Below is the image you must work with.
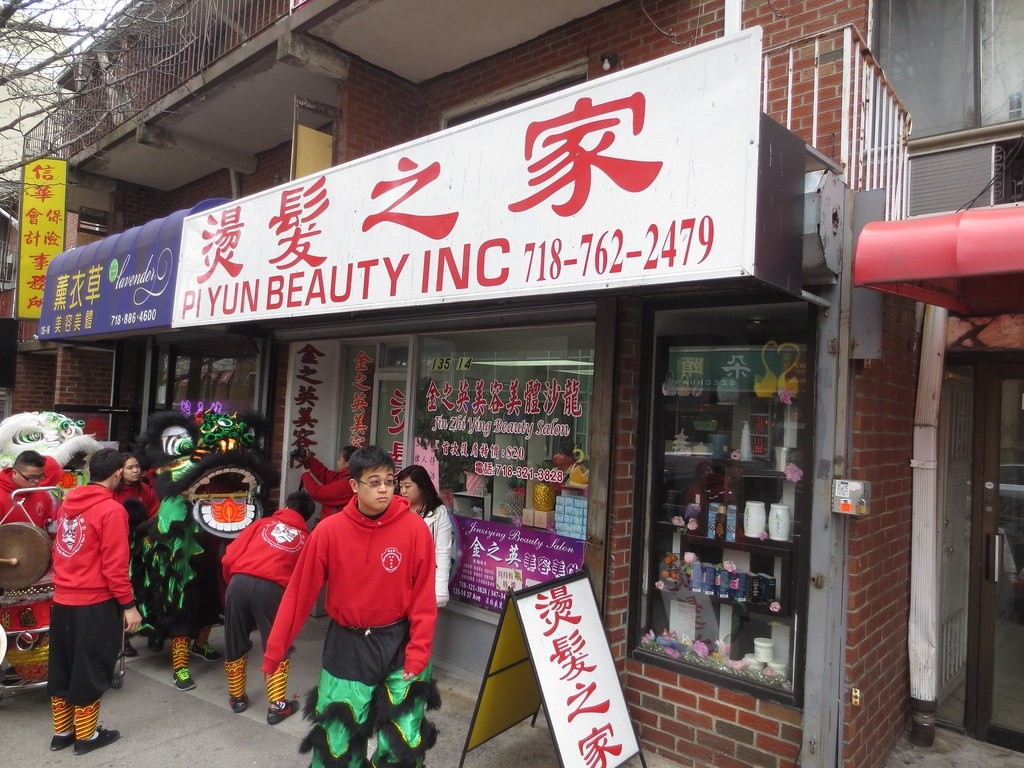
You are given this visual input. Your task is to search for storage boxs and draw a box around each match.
[523,496,588,540]
[690,560,775,606]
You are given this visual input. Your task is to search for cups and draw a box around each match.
[773,446,795,473]
[708,433,729,460]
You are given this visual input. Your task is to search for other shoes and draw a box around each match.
[122,642,138,657]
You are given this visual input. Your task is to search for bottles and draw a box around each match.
[743,501,766,538]
[740,420,752,462]
[744,638,787,678]
[768,503,791,540]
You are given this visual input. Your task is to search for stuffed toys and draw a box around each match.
[129,409,280,653]
[0,411,103,468]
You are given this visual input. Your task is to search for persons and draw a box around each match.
[0,450,53,528]
[50,448,143,755]
[221,491,316,723]
[260,445,443,768]
[169,625,223,690]
[301,446,358,521]
[113,452,151,505]
[686,447,746,563]
[397,465,452,607]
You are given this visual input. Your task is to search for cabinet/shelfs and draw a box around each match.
[453,430,590,525]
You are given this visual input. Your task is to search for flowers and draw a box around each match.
[671,513,697,534]
[759,532,768,541]
[764,667,775,677]
[731,449,740,460]
[770,601,781,612]
[654,388,801,685]
[785,462,803,482]
[654,552,736,592]
[731,659,749,671]
[639,627,708,659]
[778,388,793,405]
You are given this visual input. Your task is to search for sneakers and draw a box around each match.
[74,725,120,754]
[172,667,196,690]
[191,642,223,661]
[229,693,249,713]
[267,700,299,725]
[51,725,76,751]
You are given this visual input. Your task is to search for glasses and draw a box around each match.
[14,468,45,483]
[355,478,399,489]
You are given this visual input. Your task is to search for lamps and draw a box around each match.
[600,53,617,70]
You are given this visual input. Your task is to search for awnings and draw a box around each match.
[853,206,1024,317]
